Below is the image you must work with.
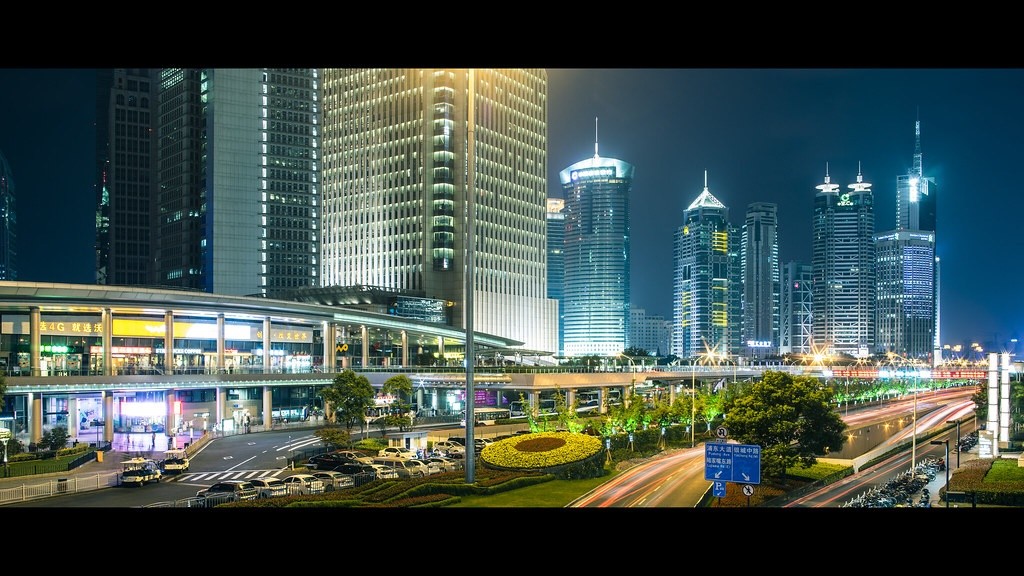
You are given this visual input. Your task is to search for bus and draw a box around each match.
[363,404,419,425]
[459,408,511,428]
[510,400,557,416]
[580,391,620,406]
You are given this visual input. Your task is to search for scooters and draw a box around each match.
[843,457,946,507]
[955,424,986,453]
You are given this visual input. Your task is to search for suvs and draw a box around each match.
[196,480,258,507]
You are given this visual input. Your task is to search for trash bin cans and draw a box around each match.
[94,451,104,463]
[57,478,68,493]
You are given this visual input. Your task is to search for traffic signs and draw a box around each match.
[705,443,761,483]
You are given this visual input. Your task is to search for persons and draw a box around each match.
[431,407,434,417]
[152,432,156,446]
[247,416,250,433]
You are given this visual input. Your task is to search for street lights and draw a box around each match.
[720,355,736,383]
[887,352,917,478]
[692,352,714,448]
[930,440,949,508]
[946,420,959,468]
[846,360,862,416]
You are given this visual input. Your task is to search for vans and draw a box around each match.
[372,457,421,479]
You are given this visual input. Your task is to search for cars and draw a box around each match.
[447,437,485,452]
[339,451,374,465]
[474,438,494,445]
[283,474,325,495]
[410,459,441,477]
[307,453,359,471]
[378,447,419,460]
[333,465,377,486]
[248,477,292,498]
[434,441,465,455]
[313,471,355,493]
[430,457,463,472]
[370,464,400,479]
